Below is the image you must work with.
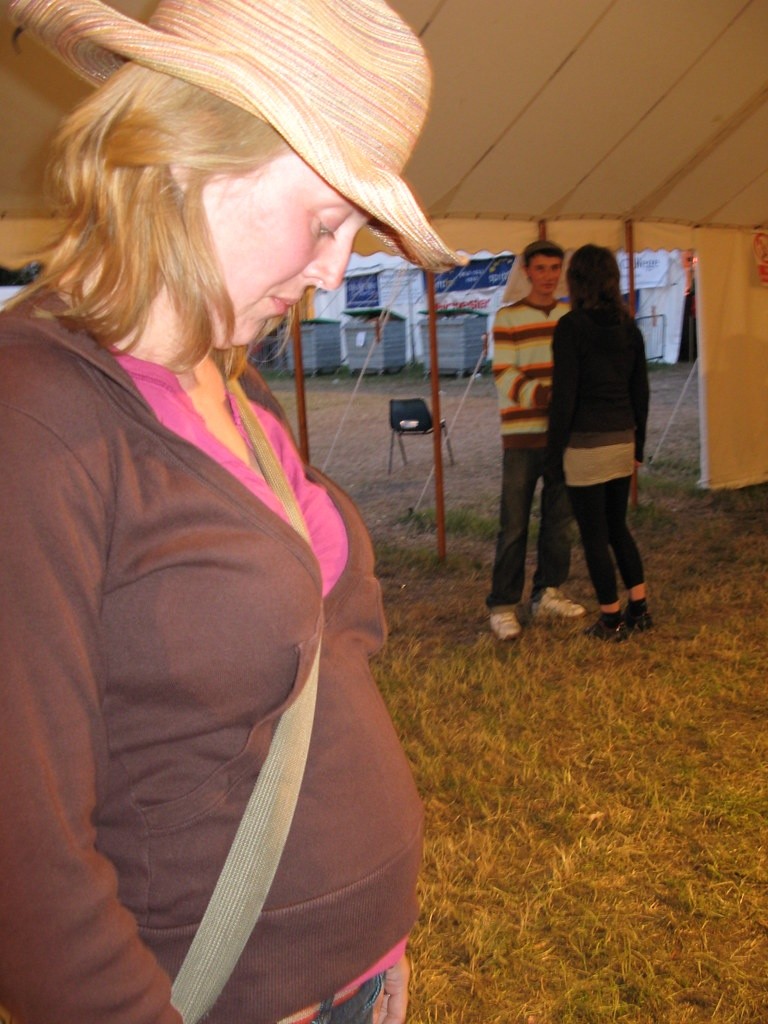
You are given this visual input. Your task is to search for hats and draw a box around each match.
[9,0,471,276]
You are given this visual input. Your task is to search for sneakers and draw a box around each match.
[531,588,585,620]
[491,613,521,639]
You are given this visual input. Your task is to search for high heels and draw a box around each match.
[587,619,628,644]
[622,610,652,630]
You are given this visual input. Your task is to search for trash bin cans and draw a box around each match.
[286,319,341,377]
[342,307,407,375]
[418,308,489,379]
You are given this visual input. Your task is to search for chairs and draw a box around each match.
[389,398,455,472]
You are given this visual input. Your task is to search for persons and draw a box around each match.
[1,1,469,1024]
[485,238,651,643]
